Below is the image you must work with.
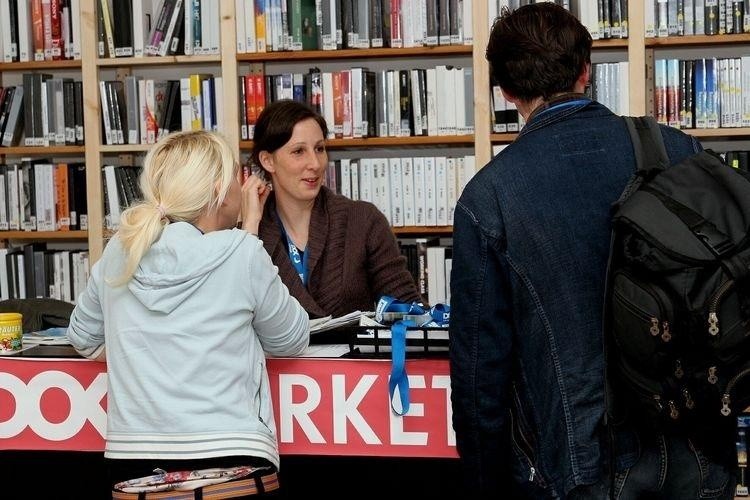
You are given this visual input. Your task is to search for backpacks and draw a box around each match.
[606,115,750,435]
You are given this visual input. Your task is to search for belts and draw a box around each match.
[111,471,280,500]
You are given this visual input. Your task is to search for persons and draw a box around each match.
[65,131,311,500]
[449,1,749,500]
[236,99,432,320]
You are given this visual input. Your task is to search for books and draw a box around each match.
[400,234,454,310]
[237,63,475,139]
[0,239,90,299]
[585,59,630,119]
[232,0,473,53]
[654,55,750,129]
[322,158,475,225]
[18,326,69,347]
[0,69,84,145]
[573,0,628,40]
[94,1,220,57]
[489,71,527,133]
[98,72,222,144]
[0,153,87,232]
[0,0,82,61]
[487,0,571,40]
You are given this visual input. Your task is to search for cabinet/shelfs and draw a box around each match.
[0,0,750,279]
